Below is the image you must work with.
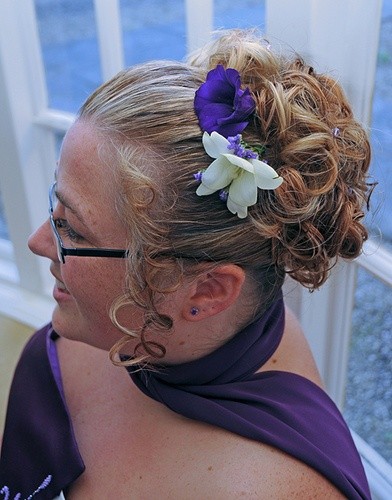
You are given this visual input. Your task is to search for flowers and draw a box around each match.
[193,64,260,137]
[195,130,284,219]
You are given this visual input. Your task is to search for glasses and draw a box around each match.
[48,181,230,265]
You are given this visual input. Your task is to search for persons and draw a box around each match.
[0,22,383,500]
